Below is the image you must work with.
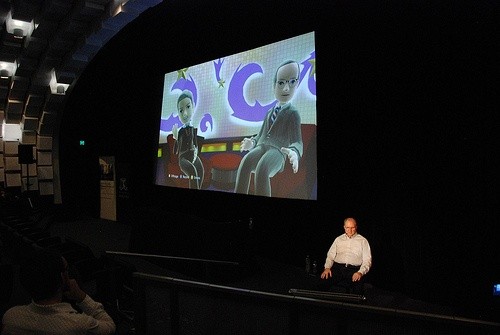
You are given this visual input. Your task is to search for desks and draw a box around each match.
[209,153,243,183]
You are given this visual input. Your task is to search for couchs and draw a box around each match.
[166,134,211,189]
[248,124,317,199]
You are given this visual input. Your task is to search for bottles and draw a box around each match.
[313,261,317,273]
[305,255,311,273]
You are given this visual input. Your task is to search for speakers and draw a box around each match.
[18,144,33,164]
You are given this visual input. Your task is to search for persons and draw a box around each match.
[319,218,372,293]
[2,240,116,335]
